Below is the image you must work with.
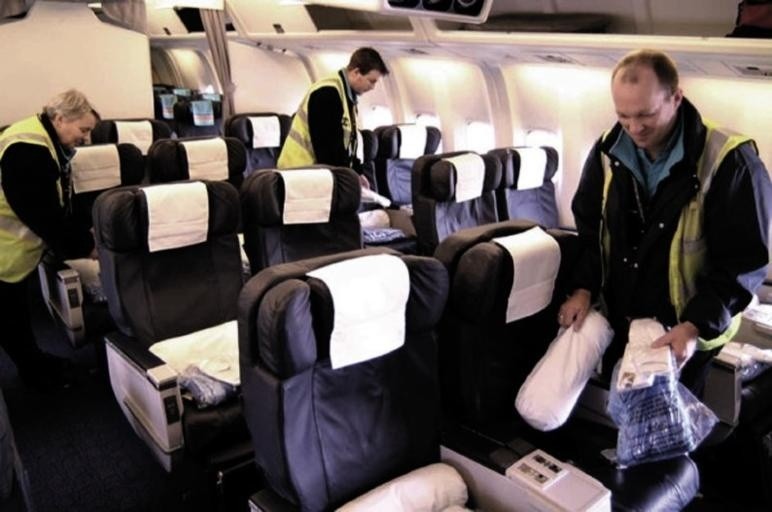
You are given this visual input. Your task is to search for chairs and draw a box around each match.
[89,181,284,479]
[412,149,501,246]
[234,247,582,511]
[36,84,443,340]
[437,217,715,483]
[494,144,567,226]
[701,316,772,429]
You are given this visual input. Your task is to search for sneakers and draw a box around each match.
[21,359,82,395]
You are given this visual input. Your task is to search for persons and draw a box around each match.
[555,49,770,473]
[0,89,96,396]
[277,45,390,199]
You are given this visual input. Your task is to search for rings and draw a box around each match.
[558,313,563,319]
